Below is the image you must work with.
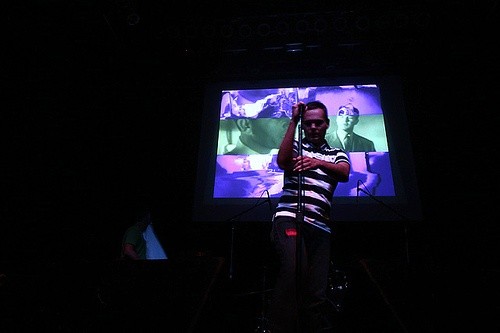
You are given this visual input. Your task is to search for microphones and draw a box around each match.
[356,180,360,203]
[299,104,305,113]
[267,192,273,208]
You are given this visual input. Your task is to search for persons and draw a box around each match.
[264,101,352,333]
[224,117,293,155]
[324,102,376,152]
[121,208,153,261]
